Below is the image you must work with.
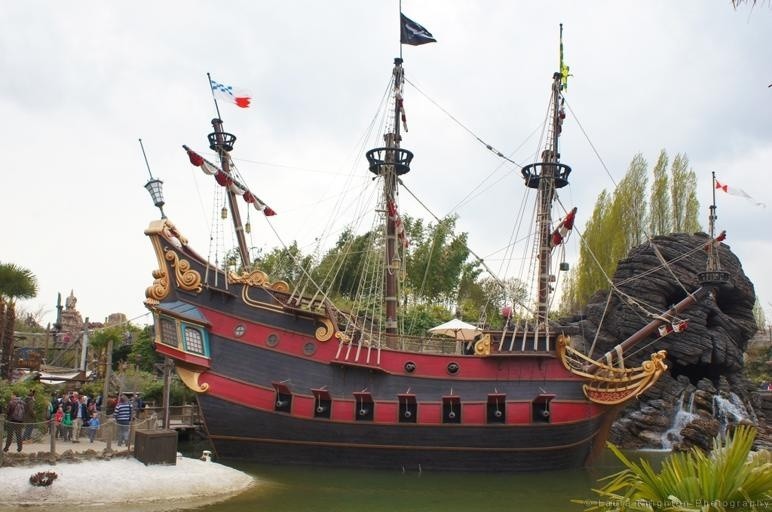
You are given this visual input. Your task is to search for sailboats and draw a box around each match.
[135,0,737,478]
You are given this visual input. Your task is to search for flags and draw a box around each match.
[560,23,569,90]
[400,13,437,47]
[210,81,255,109]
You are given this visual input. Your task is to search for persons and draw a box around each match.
[133,392,146,420]
[47,390,104,443]
[4,391,30,452]
[113,395,135,449]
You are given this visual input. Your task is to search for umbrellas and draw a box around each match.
[425,319,484,354]
